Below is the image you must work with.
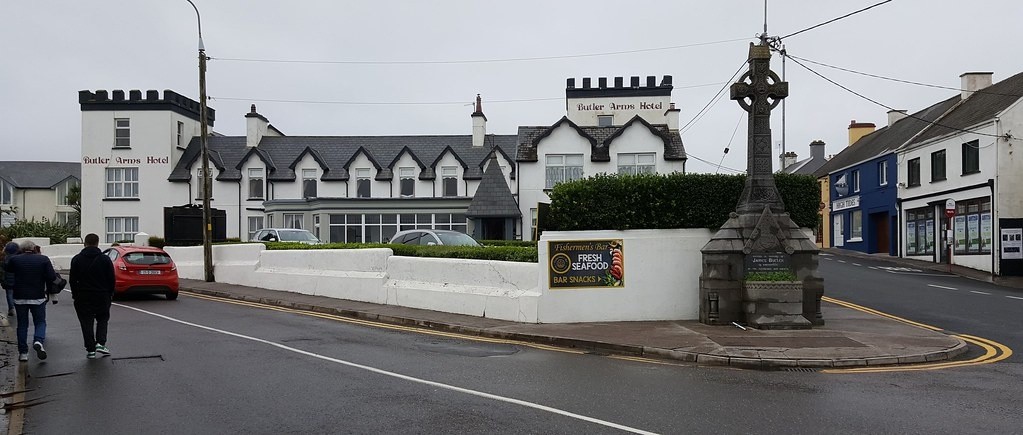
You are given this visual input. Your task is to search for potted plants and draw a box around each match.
[742,271,803,303]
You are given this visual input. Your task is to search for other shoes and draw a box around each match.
[7,309,15,316]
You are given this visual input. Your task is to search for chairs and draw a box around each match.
[142,253,154,263]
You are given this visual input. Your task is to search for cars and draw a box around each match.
[389,229,482,246]
[102,246,180,301]
[250,228,321,244]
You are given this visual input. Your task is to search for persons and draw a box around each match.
[69,234,115,358]
[0,241,58,360]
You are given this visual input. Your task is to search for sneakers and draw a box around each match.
[95,343,111,354]
[87,352,95,358]
[19,353,28,360]
[33,340,47,360]
